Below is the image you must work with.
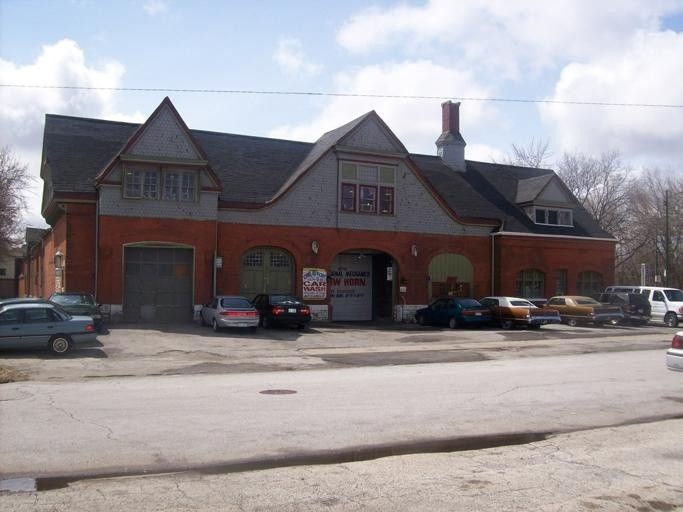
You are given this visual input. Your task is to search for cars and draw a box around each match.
[0,291,104,355]
[666,331,683,372]
[414,286,683,330]
[200,293,312,333]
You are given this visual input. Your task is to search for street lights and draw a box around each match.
[648,217,661,287]
